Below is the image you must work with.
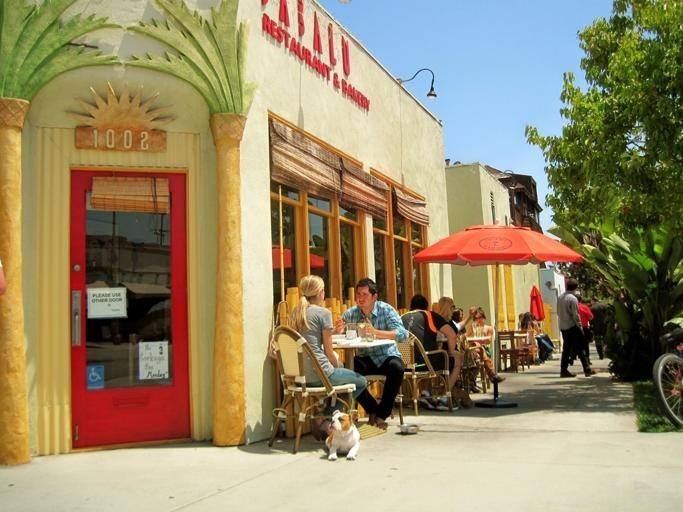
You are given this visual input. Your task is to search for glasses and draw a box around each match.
[475,316,484,321]
[451,306,456,310]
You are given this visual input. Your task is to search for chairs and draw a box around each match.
[363,328,495,425]
[268,324,356,454]
[498,329,539,373]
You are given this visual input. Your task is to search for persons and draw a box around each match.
[401,295,506,412]
[556,278,597,377]
[336,277,407,428]
[574,293,594,365]
[289,275,367,439]
[590,295,607,360]
[518,311,556,362]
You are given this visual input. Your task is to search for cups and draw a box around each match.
[345,323,356,339]
[356,323,376,342]
[471,322,484,337]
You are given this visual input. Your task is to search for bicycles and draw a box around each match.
[652,327,683,428]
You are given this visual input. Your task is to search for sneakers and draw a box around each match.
[490,374,506,383]
[436,400,459,410]
[419,394,434,409]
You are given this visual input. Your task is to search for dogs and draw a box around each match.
[324,409,361,461]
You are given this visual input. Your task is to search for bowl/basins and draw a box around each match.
[398,424,421,434]
[330,334,345,344]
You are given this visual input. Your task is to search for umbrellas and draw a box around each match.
[273,244,341,272]
[530,285,546,321]
[412,220,583,409]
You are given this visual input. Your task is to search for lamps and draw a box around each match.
[398,68,437,99]
[501,170,516,189]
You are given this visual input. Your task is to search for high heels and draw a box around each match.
[468,382,480,395]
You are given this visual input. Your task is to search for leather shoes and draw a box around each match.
[560,372,577,377]
[375,417,388,428]
[585,369,600,376]
[367,413,375,425]
